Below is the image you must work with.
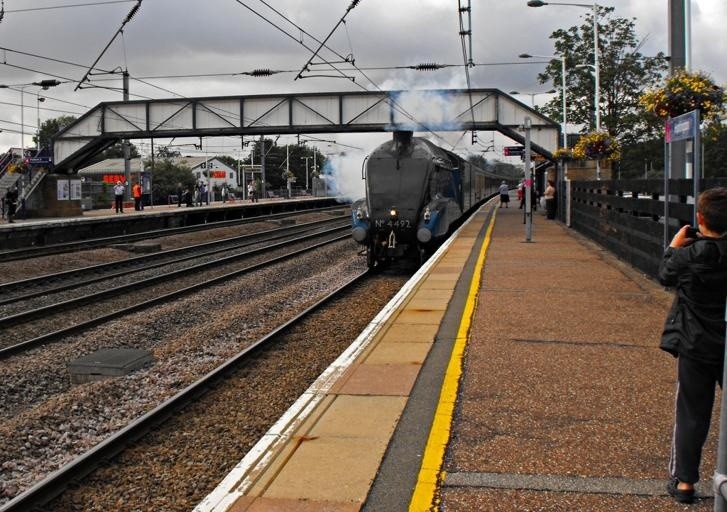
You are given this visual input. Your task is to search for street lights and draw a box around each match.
[37,86,50,150]
[300,156,313,189]
[529,0,601,179]
[508,89,557,110]
[521,53,568,173]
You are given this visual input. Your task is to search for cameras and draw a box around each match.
[118,184,120,186]
[687,228,700,238]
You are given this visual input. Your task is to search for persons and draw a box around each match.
[654,186,727,505]
[177,178,257,208]
[112,180,126,214]
[5,162,29,224]
[498,179,555,220]
[131,182,143,211]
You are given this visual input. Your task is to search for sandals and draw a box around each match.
[666,477,694,503]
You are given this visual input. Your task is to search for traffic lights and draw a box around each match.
[504,147,510,156]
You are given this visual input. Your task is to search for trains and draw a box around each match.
[351,130,526,272]
